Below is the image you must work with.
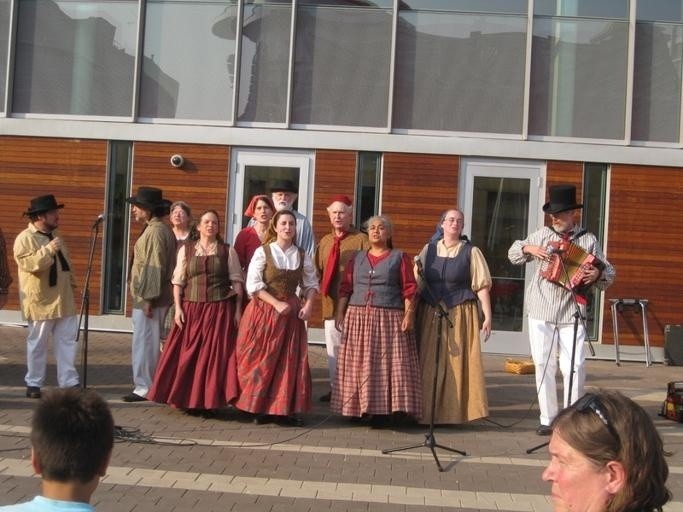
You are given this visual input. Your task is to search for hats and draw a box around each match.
[327,194,352,206]
[125,187,172,211]
[26,194,64,215]
[270,180,298,192]
[542,184,584,214]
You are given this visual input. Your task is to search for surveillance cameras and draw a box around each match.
[170,155,183,167]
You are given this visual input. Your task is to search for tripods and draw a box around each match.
[383,316,466,472]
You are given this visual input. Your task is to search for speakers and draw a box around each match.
[665,324,683,364]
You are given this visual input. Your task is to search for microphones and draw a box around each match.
[90,214,106,229]
[547,247,562,255]
[414,255,423,280]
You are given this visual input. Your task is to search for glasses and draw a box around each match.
[572,393,618,440]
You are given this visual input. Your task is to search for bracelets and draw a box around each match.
[404,308,415,313]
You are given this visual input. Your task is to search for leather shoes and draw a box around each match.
[537,425,553,435]
[121,393,147,402]
[27,387,40,398]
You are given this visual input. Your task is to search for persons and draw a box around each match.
[145,209,244,419]
[0,227,12,311]
[539,387,676,511]
[160,200,197,351]
[316,194,370,400]
[120,186,176,402]
[230,209,320,424]
[505,207,617,435]
[0,384,113,511]
[411,209,493,426]
[12,195,81,398]
[233,195,277,312]
[247,180,316,333]
[327,214,420,428]
